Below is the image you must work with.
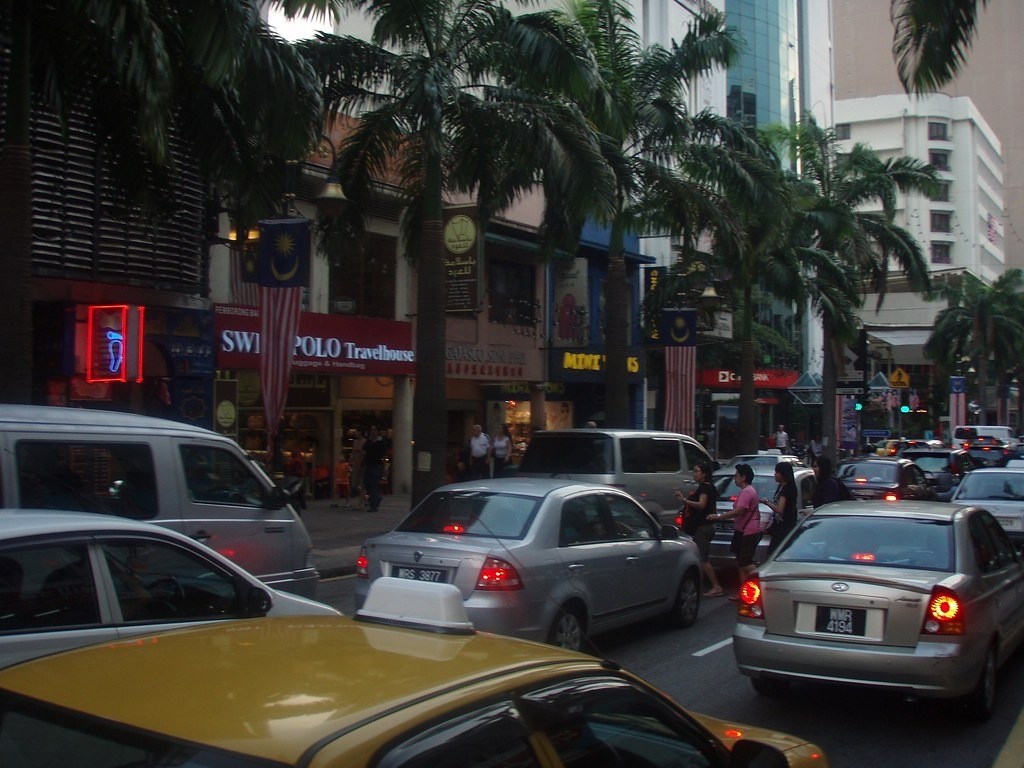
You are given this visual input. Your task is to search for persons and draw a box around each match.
[284,421,512,512]
[674,424,858,601]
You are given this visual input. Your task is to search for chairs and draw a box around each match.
[927,528,949,568]
[79,554,177,620]
[982,485,1003,496]
[840,523,881,558]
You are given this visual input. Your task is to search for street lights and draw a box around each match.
[270,133,351,467]
[676,256,726,308]
[955,361,977,424]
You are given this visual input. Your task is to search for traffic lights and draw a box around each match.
[900,389,909,413]
[855,401,863,411]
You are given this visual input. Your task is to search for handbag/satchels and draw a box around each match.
[685,516,697,536]
[730,530,743,553]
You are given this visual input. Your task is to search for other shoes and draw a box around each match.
[368,496,382,512]
[331,503,339,507]
[344,504,352,507]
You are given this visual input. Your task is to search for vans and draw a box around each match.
[952,425,1021,447]
[516,427,719,527]
[0,403,320,597]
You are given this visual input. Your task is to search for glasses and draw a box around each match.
[692,470,701,473]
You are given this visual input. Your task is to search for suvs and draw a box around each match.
[900,448,977,503]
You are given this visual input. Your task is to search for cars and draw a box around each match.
[0,505,342,669]
[835,456,939,501]
[950,467,1024,546]
[962,436,1024,468]
[677,452,818,580]
[734,499,1024,725]
[874,440,929,457]
[354,479,702,653]
[0,619,828,768]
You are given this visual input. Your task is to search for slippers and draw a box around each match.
[703,590,723,597]
